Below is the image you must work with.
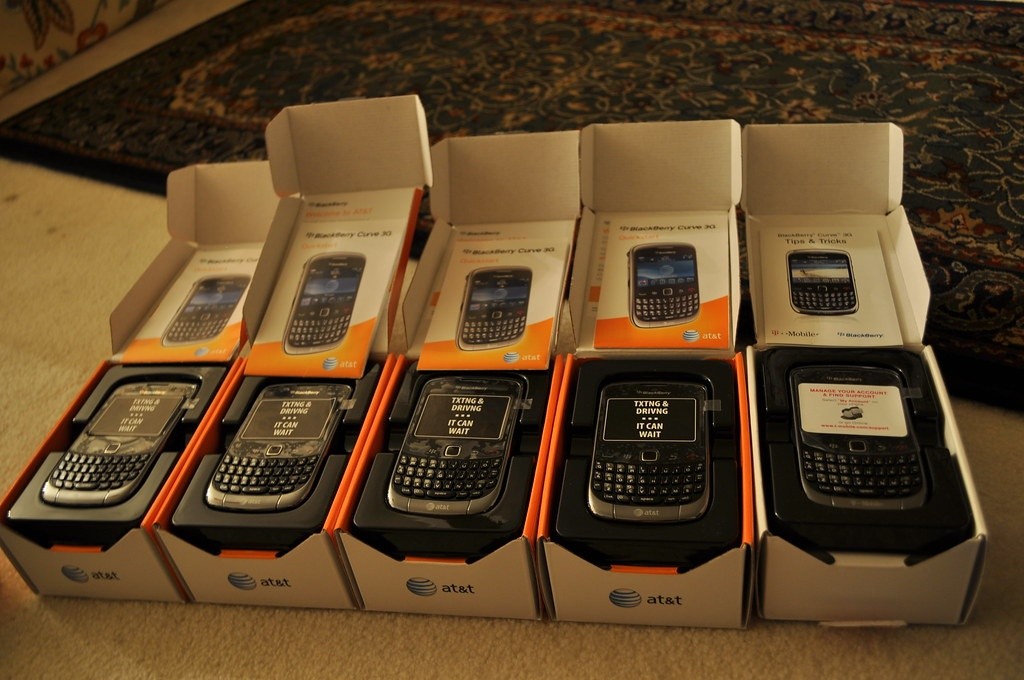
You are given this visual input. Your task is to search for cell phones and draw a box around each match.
[161,274,251,348]
[387,376,524,516]
[627,243,699,328]
[587,383,711,523]
[455,267,531,350]
[788,365,927,510]
[41,382,198,505]
[283,252,366,355]
[785,248,859,316]
[205,383,352,512]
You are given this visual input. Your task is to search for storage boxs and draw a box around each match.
[741,122,989,630]
[0,161,247,604]
[158,95,431,611]
[339,129,581,621]
[540,119,757,628]
